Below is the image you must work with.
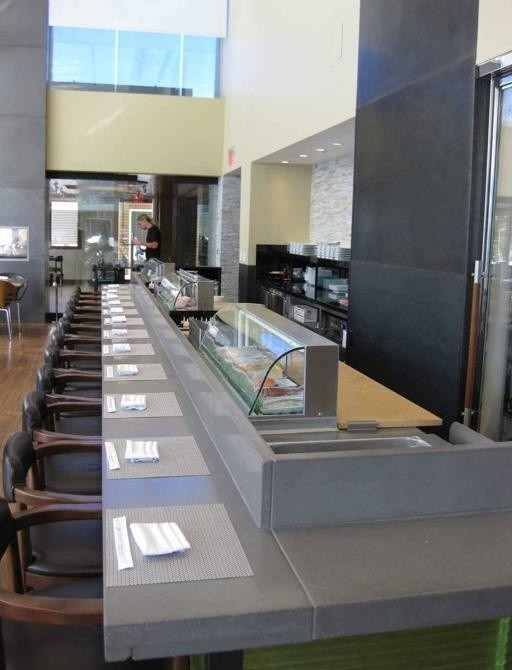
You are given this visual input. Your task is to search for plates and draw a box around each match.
[290,240,353,262]
[304,266,348,294]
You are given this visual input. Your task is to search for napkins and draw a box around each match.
[112,343,130,353]
[126,439,161,464]
[107,294,118,299]
[120,393,147,411]
[107,289,117,293]
[112,316,126,324]
[116,365,138,375]
[108,300,120,305]
[108,284,119,288]
[112,329,128,336]
[111,307,124,314]
[129,520,190,557]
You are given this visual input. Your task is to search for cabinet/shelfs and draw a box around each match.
[262,251,349,319]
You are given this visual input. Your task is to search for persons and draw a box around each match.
[131,215,162,262]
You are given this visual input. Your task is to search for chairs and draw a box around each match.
[43,343,101,417]
[3,272,28,340]
[36,365,101,437]
[59,285,101,351]
[1,280,24,340]
[46,327,101,399]
[1,432,103,591]
[1,503,190,667]
[21,393,102,496]
